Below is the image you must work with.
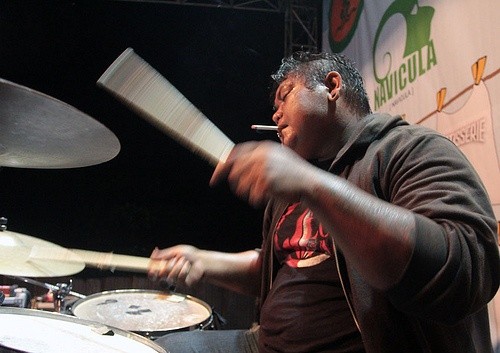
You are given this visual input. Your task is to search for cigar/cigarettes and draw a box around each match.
[251,124,280,132]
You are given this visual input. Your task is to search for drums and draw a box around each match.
[0,305,170,353]
[70,287,217,338]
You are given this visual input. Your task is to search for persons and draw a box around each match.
[145,48,500,353]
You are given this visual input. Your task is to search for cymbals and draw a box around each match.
[0,229,86,277]
[0,77,121,170]
